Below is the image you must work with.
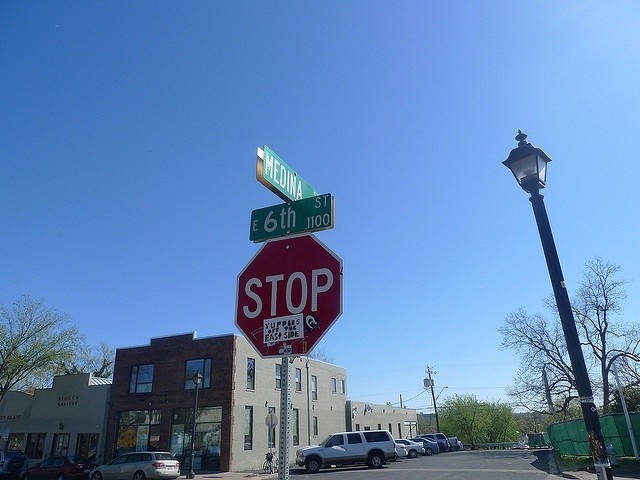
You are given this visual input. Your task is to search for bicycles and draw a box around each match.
[262,454,272,474]
[270,451,279,474]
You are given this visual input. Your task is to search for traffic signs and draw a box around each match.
[250,193,334,244]
[257,145,318,202]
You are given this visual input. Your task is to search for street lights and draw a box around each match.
[502,130,611,479]
[187,370,203,479]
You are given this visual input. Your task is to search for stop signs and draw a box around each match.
[236,233,342,360]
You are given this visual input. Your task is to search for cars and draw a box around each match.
[89,452,180,480]
[336,442,408,467]
[394,438,425,458]
[414,433,451,453]
[447,437,463,452]
[409,437,439,455]
[0,449,31,480]
[19,456,99,480]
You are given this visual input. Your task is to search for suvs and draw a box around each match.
[296,429,397,473]
